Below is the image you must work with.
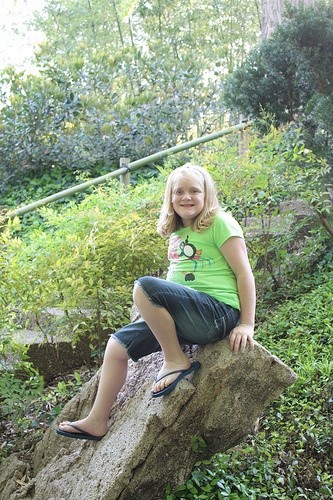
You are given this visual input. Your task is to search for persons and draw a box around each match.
[57,161,256,439]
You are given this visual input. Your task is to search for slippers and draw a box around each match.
[56,422,102,440]
[151,361,200,397]
